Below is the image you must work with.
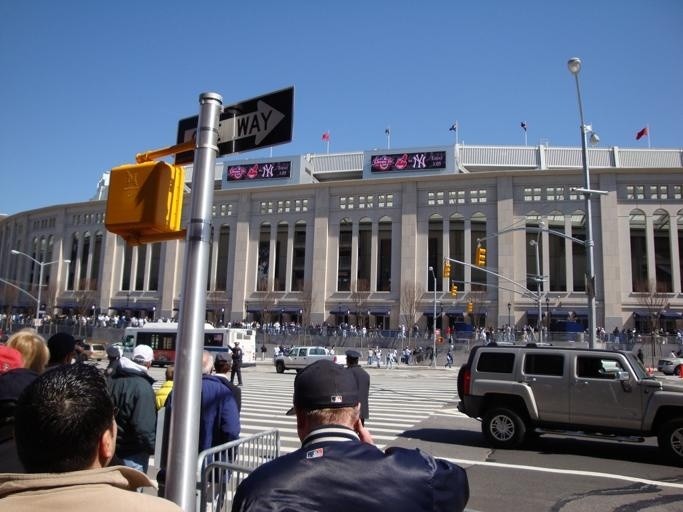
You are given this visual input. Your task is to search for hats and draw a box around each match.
[214,350,233,365]
[344,349,362,361]
[128,342,156,364]
[0,366,40,414]
[232,341,241,346]
[103,343,122,359]
[0,338,28,376]
[281,358,363,419]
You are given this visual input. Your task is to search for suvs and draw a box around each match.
[456,339,683,466]
[657,349,683,378]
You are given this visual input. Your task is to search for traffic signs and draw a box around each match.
[172,84,294,165]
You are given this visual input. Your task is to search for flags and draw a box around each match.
[322,131,329,142]
[521,121,526,132]
[584,125,592,133]
[636,127,648,140]
[385,129,390,134]
[449,124,457,132]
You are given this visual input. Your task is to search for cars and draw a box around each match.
[598,359,620,374]
[111,342,123,356]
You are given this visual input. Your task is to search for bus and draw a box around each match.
[121,320,256,371]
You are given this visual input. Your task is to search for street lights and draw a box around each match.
[125,289,132,311]
[507,301,511,327]
[9,249,72,334]
[338,300,342,322]
[529,219,547,343]
[152,306,155,320]
[90,305,95,315]
[428,266,437,367]
[568,56,602,349]
[440,302,443,329]
[545,296,550,330]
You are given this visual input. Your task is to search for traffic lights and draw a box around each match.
[466,301,474,313]
[442,262,450,278]
[450,285,458,297]
[475,246,486,269]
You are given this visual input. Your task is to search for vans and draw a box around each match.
[79,343,106,361]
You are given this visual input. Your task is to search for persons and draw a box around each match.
[425,325,453,344]
[396,324,420,339]
[244,311,384,341]
[1,313,245,512]
[595,326,683,361]
[233,343,470,512]
[473,324,550,342]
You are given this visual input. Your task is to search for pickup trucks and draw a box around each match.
[273,346,346,374]
